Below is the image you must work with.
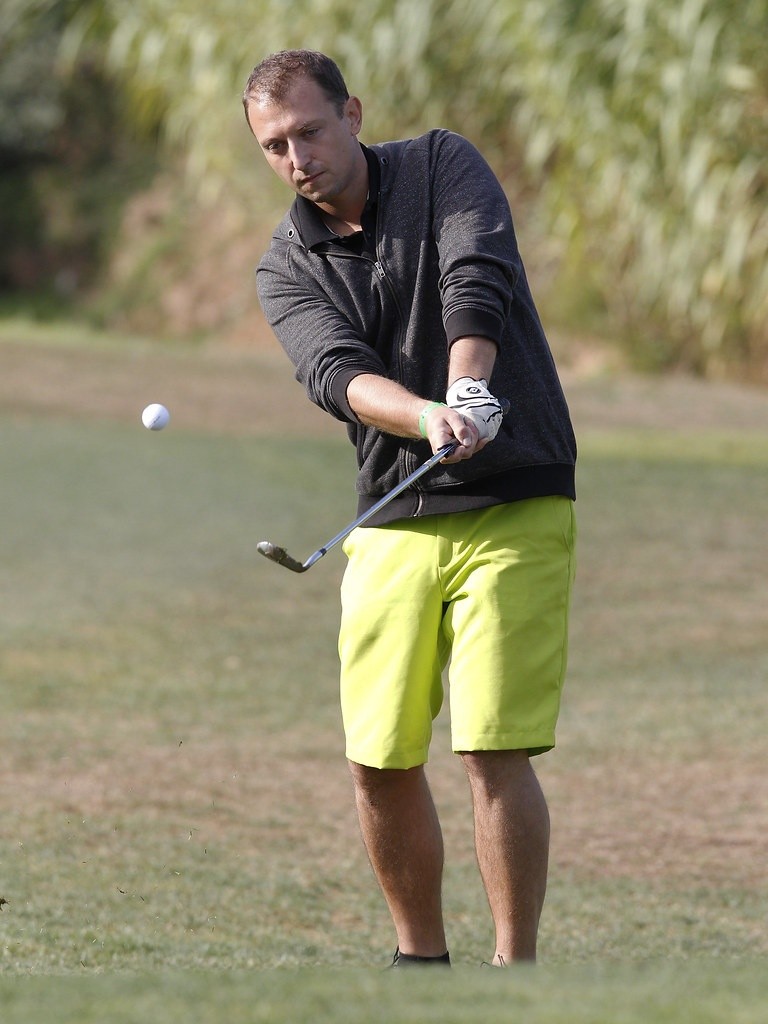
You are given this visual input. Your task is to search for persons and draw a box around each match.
[240,49,583,972]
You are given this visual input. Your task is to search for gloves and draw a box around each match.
[446,376,503,443]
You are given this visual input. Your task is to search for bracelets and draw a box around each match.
[419,400,448,438]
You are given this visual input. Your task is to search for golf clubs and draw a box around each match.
[254,437,457,573]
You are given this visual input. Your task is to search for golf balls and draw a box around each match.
[141,403,171,432]
